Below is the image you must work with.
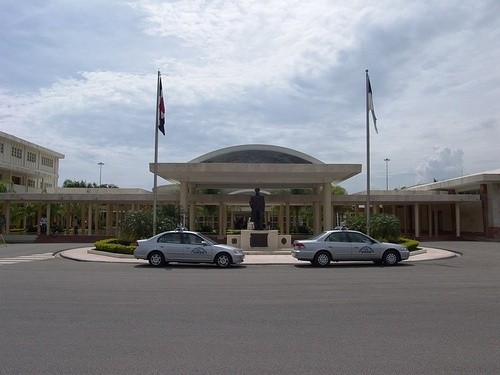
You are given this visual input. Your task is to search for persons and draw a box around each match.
[73,223,80,236]
[184,235,191,244]
[356,226,360,231]
[341,226,347,230]
[249,188,265,230]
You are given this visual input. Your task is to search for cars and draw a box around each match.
[291,222,409,267]
[131,224,245,269]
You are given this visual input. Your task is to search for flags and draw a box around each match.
[367,75,379,134]
[158,76,166,136]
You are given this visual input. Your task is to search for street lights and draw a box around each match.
[97,162,104,187]
[384,158,391,192]
